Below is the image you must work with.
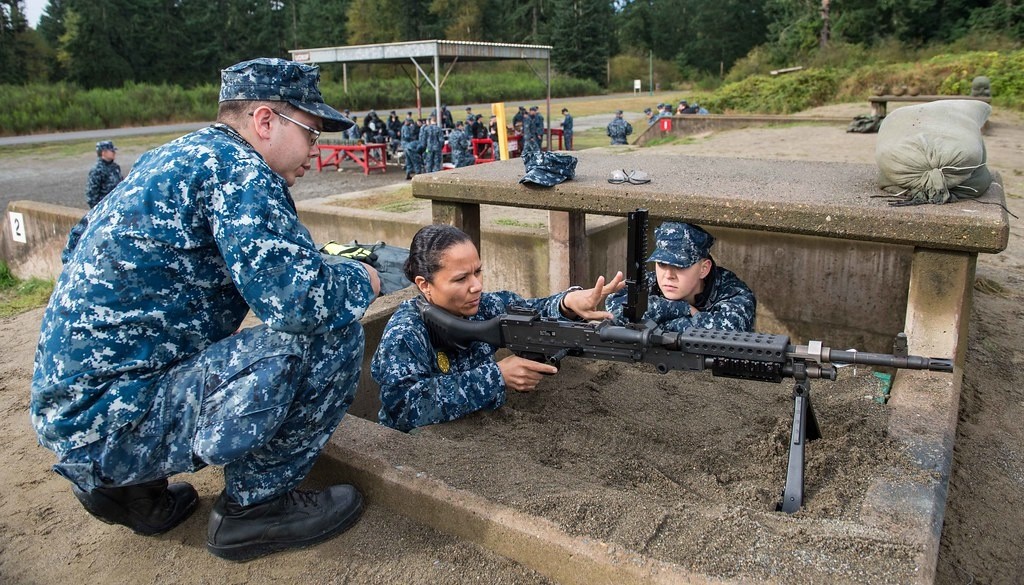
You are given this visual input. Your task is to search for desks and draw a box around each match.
[411,150,1010,363]
[471,135,522,165]
[544,128,574,151]
[317,143,387,176]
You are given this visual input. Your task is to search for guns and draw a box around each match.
[421,207,955,515]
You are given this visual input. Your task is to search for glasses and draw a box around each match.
[608,169,651,185]
[249,109,321,146]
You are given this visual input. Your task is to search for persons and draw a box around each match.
[644,103,673,126]
[86,141,124,209]
[30,58,381,564]
[677,101,708,115]
[605,222,757,333]
[513,107,545,156]
[560,108,573,151]
[371,226,629,432]
[607,110,633,146]
[341,106,500,180]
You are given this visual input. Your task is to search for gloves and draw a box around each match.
[324,242,379,265]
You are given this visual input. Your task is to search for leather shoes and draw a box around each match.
[208,484,366,562]
[71,479,199,536]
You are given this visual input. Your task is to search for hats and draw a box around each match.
[96,141,118,151]
[218,57,356,132]
[519,150,577,187]
[645,222,717,268]
[403,104,572,127]
[615,101,701,119]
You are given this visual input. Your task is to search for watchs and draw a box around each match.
[562,286,584,313]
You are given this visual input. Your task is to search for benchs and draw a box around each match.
[867,95,992,136]
